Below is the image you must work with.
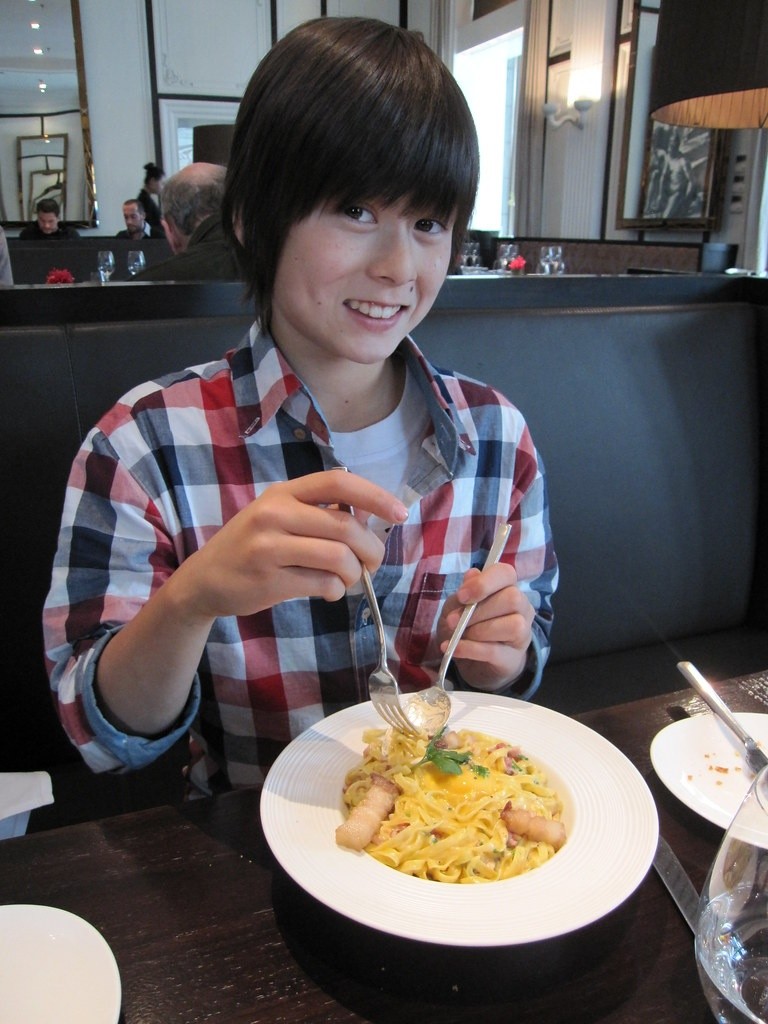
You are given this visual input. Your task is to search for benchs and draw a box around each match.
[0,237,768,837]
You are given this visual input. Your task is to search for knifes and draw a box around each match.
[676,660,768,774]
[652,835,701,939]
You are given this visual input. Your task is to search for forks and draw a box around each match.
[331,466,423,739]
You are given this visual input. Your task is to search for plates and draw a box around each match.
[258,690,660,948]
[0,903,123,1024]
[649,711,768,852]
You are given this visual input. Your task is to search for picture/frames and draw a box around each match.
[614,1,731,231]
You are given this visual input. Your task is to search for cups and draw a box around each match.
[453,239,566,277]
[127,251,146,276]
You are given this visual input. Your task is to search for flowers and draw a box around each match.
[48,267,74,284]
[509,256,525,270]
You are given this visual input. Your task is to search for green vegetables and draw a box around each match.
[413,725,529,777]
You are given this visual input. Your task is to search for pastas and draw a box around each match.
[342,724,564,883]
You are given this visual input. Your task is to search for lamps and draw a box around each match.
[647,0,768,130]
[542,0,609,129]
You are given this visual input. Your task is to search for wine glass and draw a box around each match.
[97,251,116,282]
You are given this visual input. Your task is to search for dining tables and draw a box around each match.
[0,669,768,1024]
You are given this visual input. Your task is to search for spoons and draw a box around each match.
[399,523,514,734]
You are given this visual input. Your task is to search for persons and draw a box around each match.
[116,199,165,238]
[0,226,15,285]
[38,17,558,802]
[127,162,253,282]
[138,162,166,232]
[656,134,693,218]
[19,199,80,240]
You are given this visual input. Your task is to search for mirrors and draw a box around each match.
[0,0,98,229]
